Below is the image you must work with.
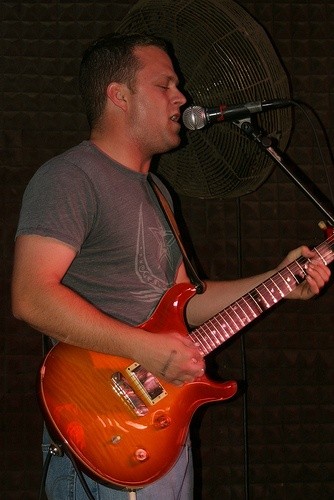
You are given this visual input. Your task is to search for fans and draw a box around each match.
[115,0,292,199]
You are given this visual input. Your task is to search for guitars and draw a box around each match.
[40,227,334,493]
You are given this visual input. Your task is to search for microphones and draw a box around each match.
[182,97,290,131]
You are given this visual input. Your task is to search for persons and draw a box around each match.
[9,31,331,500]
